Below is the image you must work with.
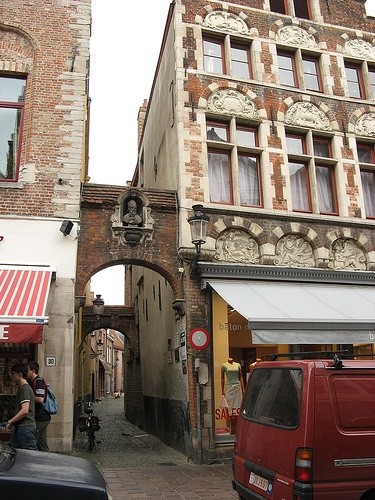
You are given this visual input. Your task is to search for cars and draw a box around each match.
[0,440,112,500]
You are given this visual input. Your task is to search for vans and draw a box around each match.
[232,349,375,500]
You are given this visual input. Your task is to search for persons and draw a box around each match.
[5,361,49,452]
[221,359,261,417]
[123,200,142,228]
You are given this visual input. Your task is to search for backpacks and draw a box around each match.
[34,378,58,414]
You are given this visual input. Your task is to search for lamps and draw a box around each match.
[187,204,210,281]
[59,219,74,236]
[90,339,104,359]
[82,295,104,330]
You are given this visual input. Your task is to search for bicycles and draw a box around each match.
[77,398,103,451]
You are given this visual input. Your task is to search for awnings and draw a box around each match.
[0,266,53,343]
[208,282,375,343]
